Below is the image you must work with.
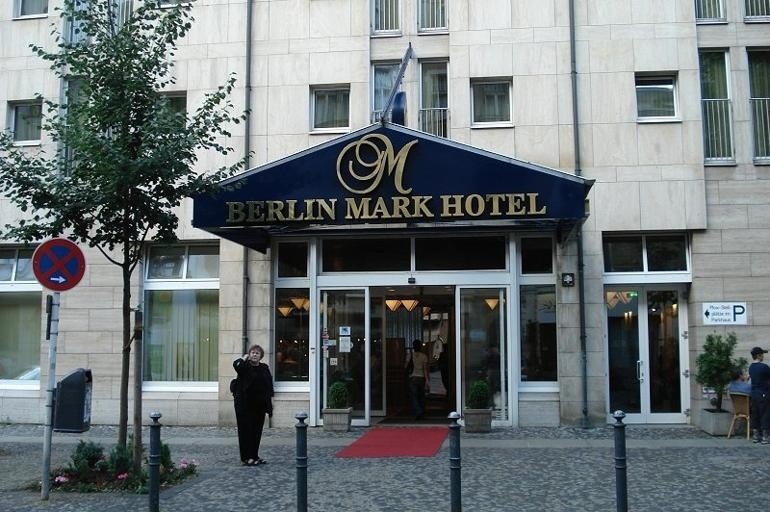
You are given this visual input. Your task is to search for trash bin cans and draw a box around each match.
[51,367,94,435]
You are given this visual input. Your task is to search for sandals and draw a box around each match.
[258,457,269,464]
[241,459,260,466]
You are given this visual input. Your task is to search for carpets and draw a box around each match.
[334,424,451,460]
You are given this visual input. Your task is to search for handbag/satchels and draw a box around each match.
[404,360,415,376]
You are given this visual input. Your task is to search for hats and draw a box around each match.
[751,347,768,354]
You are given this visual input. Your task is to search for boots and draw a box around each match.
[752,428,760,442]
[761,429,770,444]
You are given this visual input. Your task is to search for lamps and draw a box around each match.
[277,288,499,319]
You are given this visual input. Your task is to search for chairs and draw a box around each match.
[726,390,754,439]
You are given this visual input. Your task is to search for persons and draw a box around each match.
[748,346,770,444]
[728,366,753,398]
[232,345,276,467]
[403,339,430,421]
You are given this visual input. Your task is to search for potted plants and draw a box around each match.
[693,330,750,439]
[321,381,355,431]
[462,379,495,434]
